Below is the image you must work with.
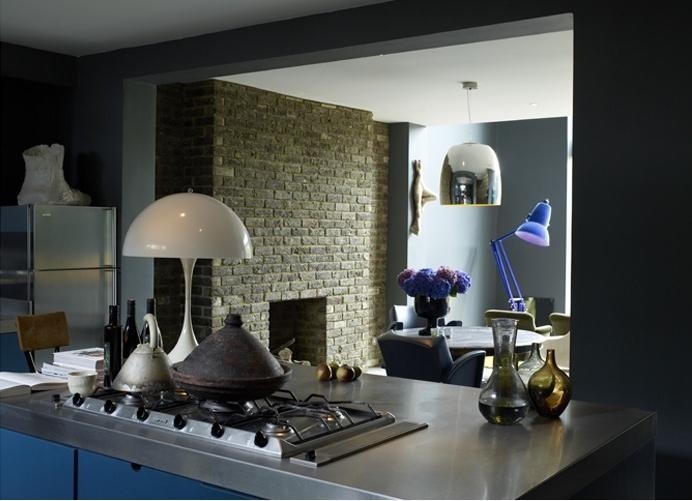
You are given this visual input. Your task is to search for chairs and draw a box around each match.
[485,309,552,340]
[16,311,70,374]
[550,313,571,337]
[378,329,486,387]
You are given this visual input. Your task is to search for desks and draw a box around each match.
[394,325,547,377]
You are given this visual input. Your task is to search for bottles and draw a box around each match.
[517,343,546,390]
[529,350,573,418]
[140,297,163,349]
[123,299,140,364]
[103,304,121,386]
[479,319,531,426]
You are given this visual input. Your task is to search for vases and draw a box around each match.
[414,297,449,336]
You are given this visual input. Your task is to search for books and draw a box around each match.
[40,346,105,383]
[0,370,69,399]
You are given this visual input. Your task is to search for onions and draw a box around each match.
[329,362,340,380]
[315,363,332,382]
[336,364,356,382]
[351,367,362,379]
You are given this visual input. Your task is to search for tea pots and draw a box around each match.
[111,314,176,393]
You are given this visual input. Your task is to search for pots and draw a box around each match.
[172,312,293,402]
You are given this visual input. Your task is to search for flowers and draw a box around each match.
[396,266,471,300]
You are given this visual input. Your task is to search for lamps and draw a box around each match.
[121,188,252,366]
[440,80,502,207]
[489,199,553,312]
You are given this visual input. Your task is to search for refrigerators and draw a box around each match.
[0,204,118,372]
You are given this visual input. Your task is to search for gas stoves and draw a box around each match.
[63,385,429,468]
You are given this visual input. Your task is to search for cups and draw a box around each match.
[68,371,98,396]
